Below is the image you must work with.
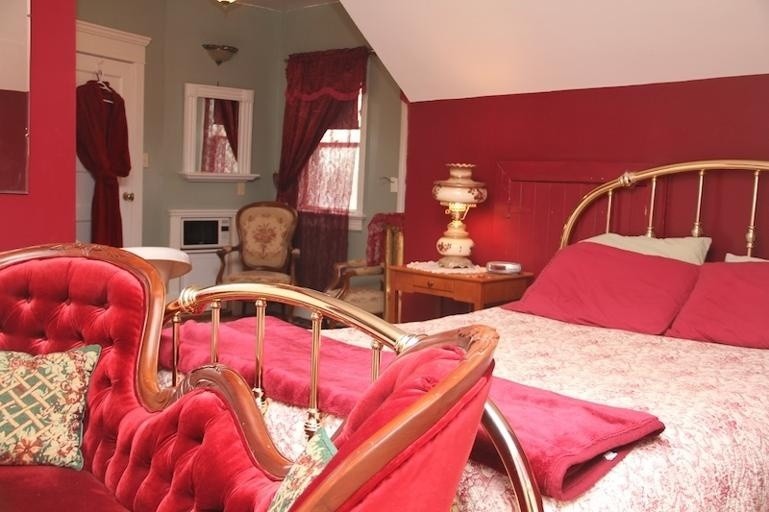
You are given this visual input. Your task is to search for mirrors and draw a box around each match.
[0,1,32,196]
[183,81,255,175]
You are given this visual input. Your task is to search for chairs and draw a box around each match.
[216,196,301,321]
[320,213,403,328]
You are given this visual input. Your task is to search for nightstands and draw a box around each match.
[387,264,534,325]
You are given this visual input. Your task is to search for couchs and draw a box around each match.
[0,239,499,512]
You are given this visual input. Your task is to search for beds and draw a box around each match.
[162,155,769,512]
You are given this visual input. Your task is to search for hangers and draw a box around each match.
[92,65,113,93]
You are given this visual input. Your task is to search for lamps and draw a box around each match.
[429,162,489,270]
[202,44,239,66]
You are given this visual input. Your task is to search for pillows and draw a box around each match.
[502,232,769,350]
[0,337,104,471]
